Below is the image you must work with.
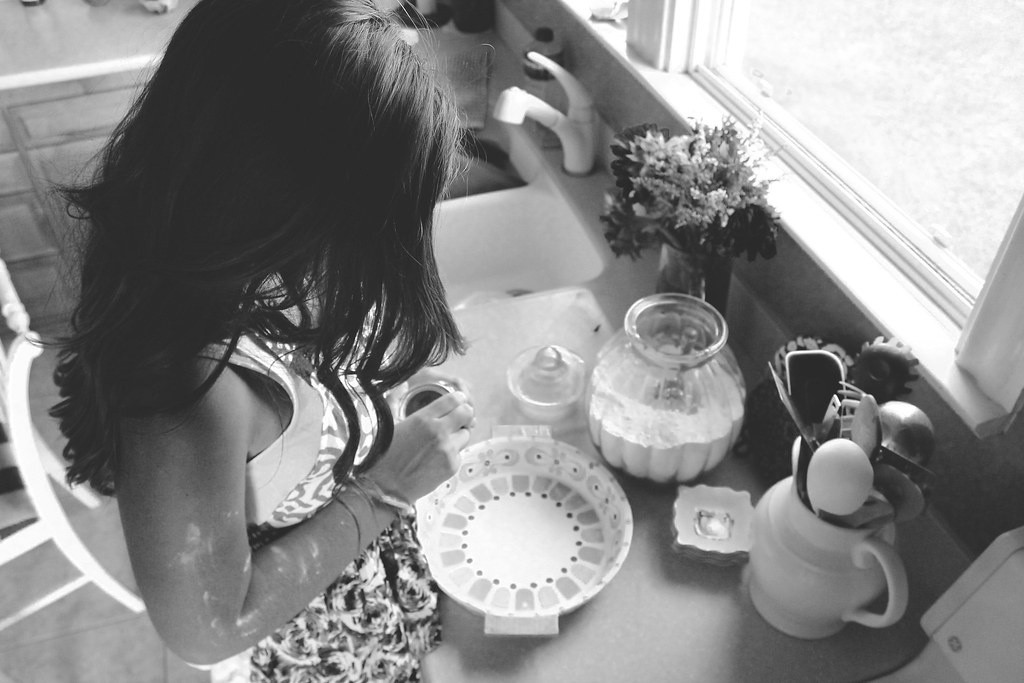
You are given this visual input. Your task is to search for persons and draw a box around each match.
[26,0,479,683]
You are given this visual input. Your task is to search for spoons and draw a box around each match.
[807,393,938,528]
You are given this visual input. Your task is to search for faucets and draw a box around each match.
[491,49,597,176]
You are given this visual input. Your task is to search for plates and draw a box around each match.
[671,484,757,569]
[415,435,634,618]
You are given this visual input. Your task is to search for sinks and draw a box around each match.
[432,86,550,206]
[360,172,608,339]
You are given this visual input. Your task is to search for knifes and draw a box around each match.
[768,361,816,454]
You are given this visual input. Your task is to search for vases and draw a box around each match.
[656,241,731,318]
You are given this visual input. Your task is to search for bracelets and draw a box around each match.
[334,473,412,556]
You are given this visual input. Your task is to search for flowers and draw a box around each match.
[596,112,780,263]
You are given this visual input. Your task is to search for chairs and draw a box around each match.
[0,256,145,633]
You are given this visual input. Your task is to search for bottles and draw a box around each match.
[521,27,565,134]
[584,292,747,484]
[395,381,457,423]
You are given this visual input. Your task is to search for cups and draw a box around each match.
[506,344,584,423]
[748,474,908,641]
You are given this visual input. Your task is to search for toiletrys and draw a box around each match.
[522,27,568,151]
